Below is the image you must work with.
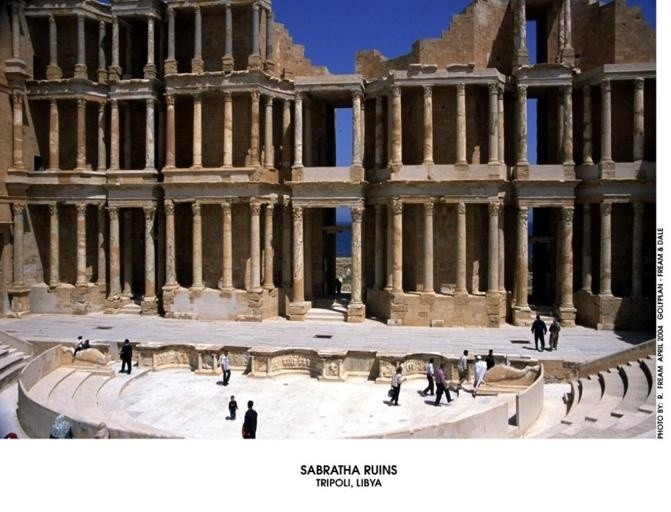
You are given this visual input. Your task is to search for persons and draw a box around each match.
[50,415,74,438]
[549,320,561,351]
[221,350,230,385]
[336,278,341,293]
[229,396,237,420]
[93,422,109,438]
[74,335,83,356]
[531,316,547,351]
[81,340,90,349]
[423,349,496,406]
[242,401,257,439]
[390,367,407,406]
[119,340,132,374]
